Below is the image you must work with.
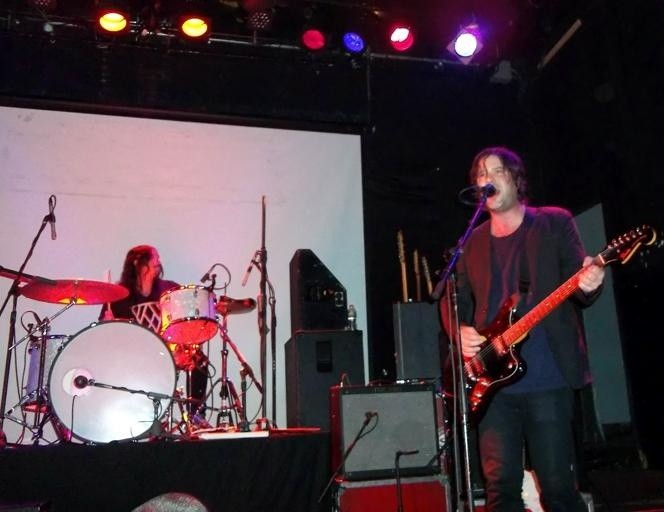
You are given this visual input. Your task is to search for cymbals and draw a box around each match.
[216,299,253,315]
[21,279,130,305]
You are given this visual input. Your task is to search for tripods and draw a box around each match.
[0,314,69,445]
[171,325,308,430]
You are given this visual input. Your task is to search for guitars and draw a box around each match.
[444,224,664,418]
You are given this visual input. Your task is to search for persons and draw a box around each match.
[94,243,229,436]
[437,145,607,511]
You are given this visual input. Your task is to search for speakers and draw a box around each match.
[288,247,348,330]
[282,332,364,426]
[394,297,445,382]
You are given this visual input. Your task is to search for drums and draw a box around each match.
[48,319,178,444]
[23,335,74,413]
[159,284,219,346]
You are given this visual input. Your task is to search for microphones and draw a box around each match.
[478,184,497,198]
[255,294,269,334]
[199,273,209,288]
[45,197,61,241]
[239,247,261,286]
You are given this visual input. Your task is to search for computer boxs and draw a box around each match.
[329,382,440,480]
